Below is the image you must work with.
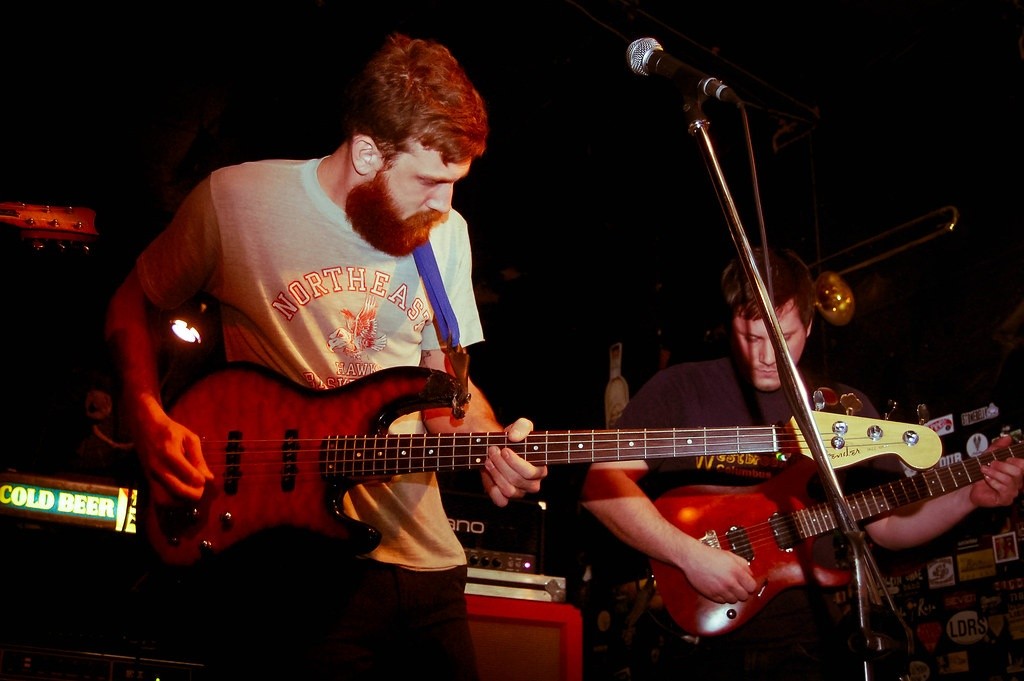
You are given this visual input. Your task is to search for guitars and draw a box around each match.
[0,201,100,255]
[648,440,1024,638]
[143,357,946,574]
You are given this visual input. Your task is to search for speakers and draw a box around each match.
[462,594,584,681]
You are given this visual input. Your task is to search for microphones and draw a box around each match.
[625,37,745,108]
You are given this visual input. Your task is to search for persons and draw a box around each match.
[106,36,548,681]
[579,245,1024,681]
